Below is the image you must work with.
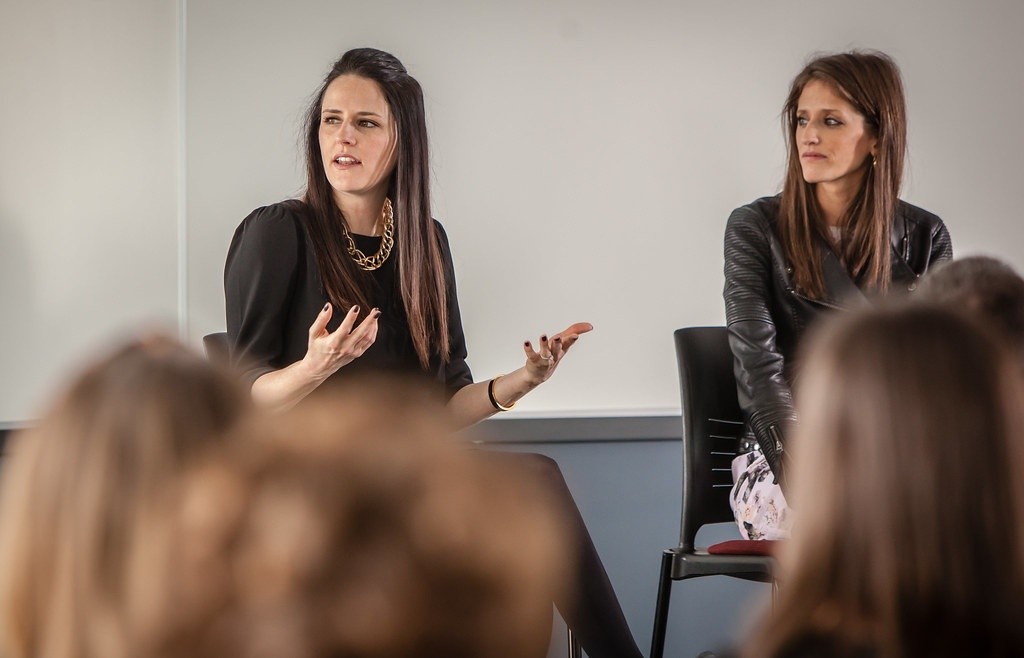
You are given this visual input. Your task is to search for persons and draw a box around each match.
[702,293,1023,658]
[723,47,955,541]
[0,337,262,657]
[905,252,1024,376]
[124,372,566,658]
[223,47,644,658]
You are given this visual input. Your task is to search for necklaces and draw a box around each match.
[341,195,396,271]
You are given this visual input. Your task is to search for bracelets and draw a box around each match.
[487,379,508,411]
[492,373,516,411]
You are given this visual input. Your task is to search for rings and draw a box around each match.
[539,350,554,361]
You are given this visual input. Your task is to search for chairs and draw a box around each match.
[648,326,780,658]
